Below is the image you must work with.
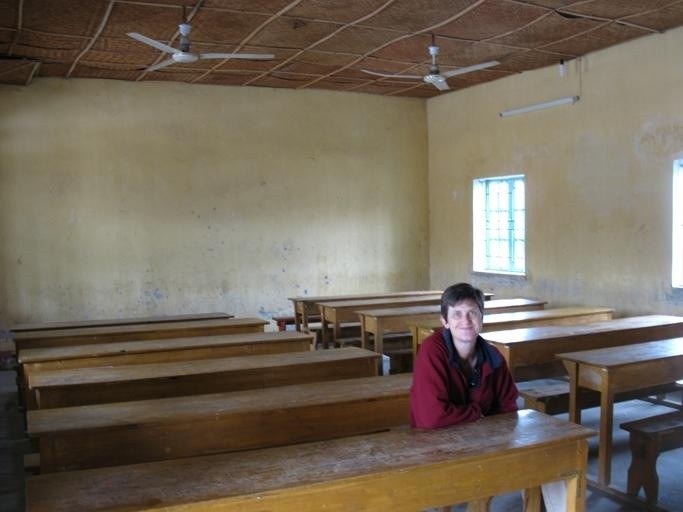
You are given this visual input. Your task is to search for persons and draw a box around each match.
[410,279,524,511]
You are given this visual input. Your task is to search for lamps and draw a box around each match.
[497,54,583,120]
[558,54,565,78]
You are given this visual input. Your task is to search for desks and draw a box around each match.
[24,408,600,511]
[354,297,548,367]
[8,317,272,353]
[28,348,384,408]
[18,328,316,412]
[406,304,617,374]
[554,336,682,491]
[9,311,233,333]
[28,373,414,473]
[478,312,682,386]
[311,294,495,348]
[288,289,445,334]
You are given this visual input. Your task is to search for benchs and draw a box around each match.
[618,410,681,505]
[518,381,682,415]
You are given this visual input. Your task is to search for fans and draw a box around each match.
[359,36,502,99]
[123,19,279,87]
